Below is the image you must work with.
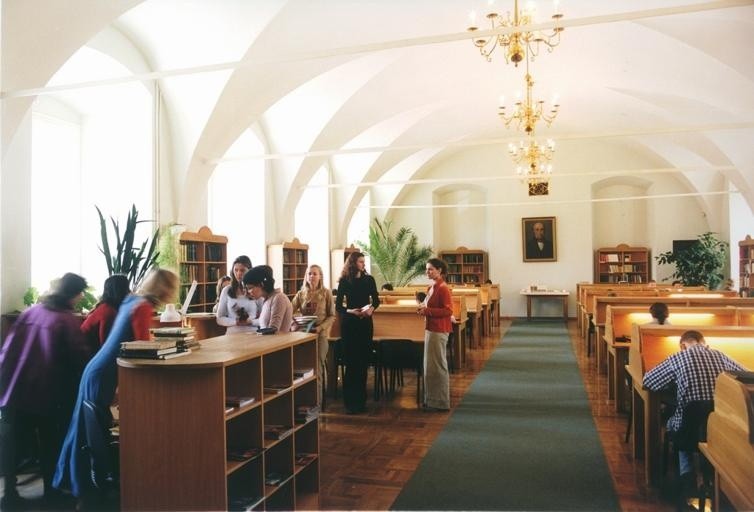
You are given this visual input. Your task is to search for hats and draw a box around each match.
[242,265,274,282]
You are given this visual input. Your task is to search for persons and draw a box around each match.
[416,292,426,304]
[641,330,750,498]
[382,284,393,291]
[485,279,492,285]
[212,254,336,413]
[79,274,130,351]
[642,302,674,325]
[52,269,179,512]
[648,280,657,288]
[672,281,681,288]
[526,222,553,258]
[415,258,454,412]
[336,252,380,414]
[0,273,91,512]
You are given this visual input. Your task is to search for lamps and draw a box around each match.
[466,0,564,191]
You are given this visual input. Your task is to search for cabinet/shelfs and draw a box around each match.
[739,240,754,288]
[177,226,228,312]
[116,331,321,512]
[595,244,648,284]
[267,239,309,296]
[438,247,489,285]
[331,244,360,289]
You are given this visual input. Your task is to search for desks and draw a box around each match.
[327,283,503,401]
[575,284,754,373]
[697,370,754,512]
[600,305,754,412]
[520,289,569,323]
[624,326,754,486]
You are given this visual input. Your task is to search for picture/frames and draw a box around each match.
[522,217,557,262]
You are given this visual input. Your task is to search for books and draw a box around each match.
[600,254,642,284]
[442,253,483,283]
[741,248,754,287]
[118,326,321,512]
[179,243,307,313]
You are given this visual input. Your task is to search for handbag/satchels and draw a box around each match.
[256,326,277,335]
